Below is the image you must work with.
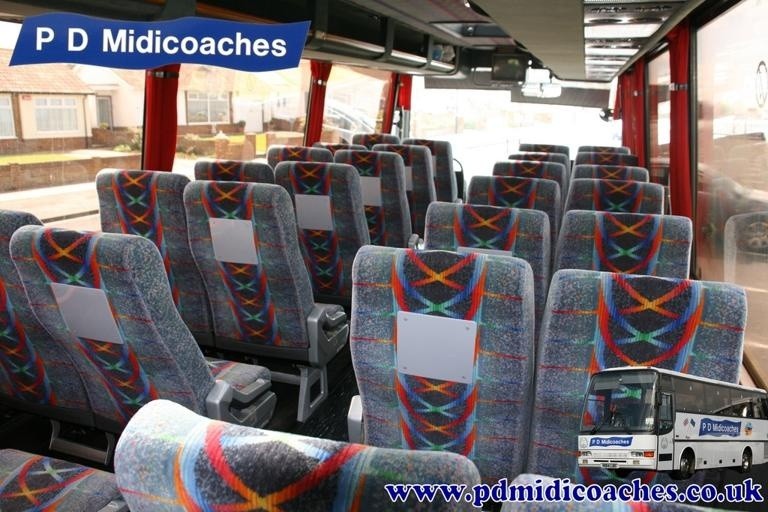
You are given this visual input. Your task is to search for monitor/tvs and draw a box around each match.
[491,51,533,82]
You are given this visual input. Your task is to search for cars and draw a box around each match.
[651,156,768,252]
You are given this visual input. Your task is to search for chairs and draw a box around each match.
[113,397,482,512]
[464,175,559,219]
[554,208,694,281]
[9,224,277,464]
[192,159,272,183]
[182,180,349,424]
[564,177,666,210]
[0,447,127,511]
[578,145,631,156]
[348,244,537,489]
[94,167,216,354]
[507,151,571,180]
[576,151,639,169]
[0,208,94,462]
[273,160,372,306]
[265,144,334,163]
[400,139,457,203]
[422,201,552,316]
[334,149,420,250]
[527,268,747,474]
[520,143,569,151]
[571,163,650,183]
[500,471,729,512]
[310,143,367,154]
[352,133,400,154]
[492,159,566,196]
[371,143,437,237]
